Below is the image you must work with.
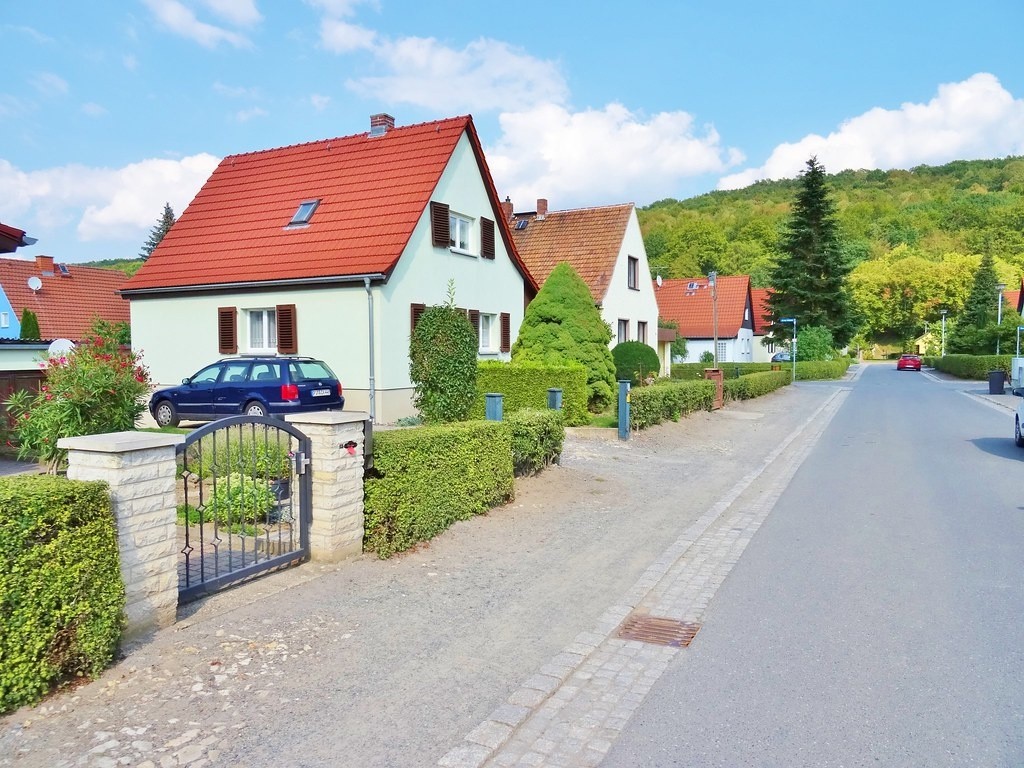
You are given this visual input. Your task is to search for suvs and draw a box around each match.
[148,347,345,430]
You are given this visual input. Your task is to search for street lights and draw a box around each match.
[940,309,949,356]
[994,282,1009,355]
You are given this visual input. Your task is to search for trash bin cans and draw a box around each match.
[988,371,1005,395]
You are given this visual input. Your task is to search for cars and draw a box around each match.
[771,352,793,362]
[896,354,921,371]
[1012,388,1024,448]
